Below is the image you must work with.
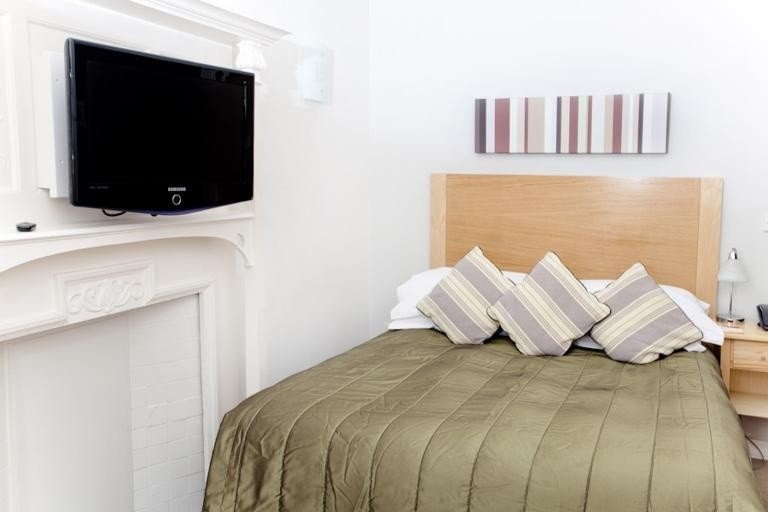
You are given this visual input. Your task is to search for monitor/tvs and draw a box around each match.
[63,36,255,217]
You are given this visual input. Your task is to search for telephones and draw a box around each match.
[756,304,768,330]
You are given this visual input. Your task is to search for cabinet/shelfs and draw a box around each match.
[717,319,768,462]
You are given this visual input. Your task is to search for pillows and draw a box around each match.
[587,262,704,363]
[487,249,611,358]
[572,280,722,353]
[416,245,515,347]
[391,266,530,331]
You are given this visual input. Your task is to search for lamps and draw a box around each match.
[717,247,743,324]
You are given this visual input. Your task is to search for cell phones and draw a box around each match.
[16,222,36,232]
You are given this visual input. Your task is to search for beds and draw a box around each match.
[223,173,755,512]
